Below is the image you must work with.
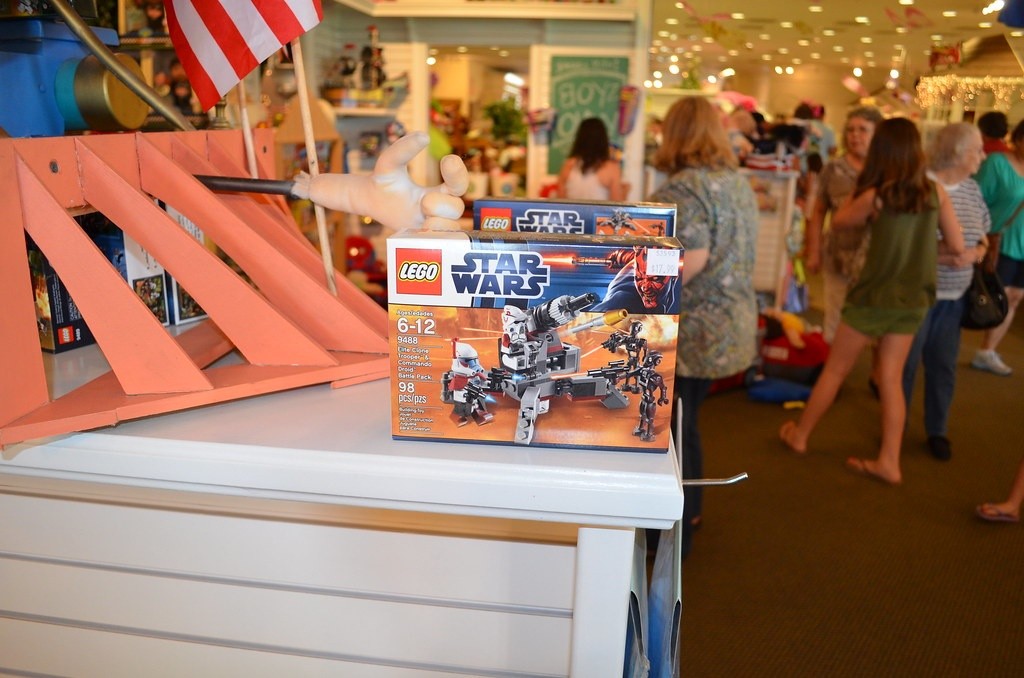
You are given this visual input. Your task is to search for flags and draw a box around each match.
[165,0,324,113]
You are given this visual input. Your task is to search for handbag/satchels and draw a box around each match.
[978,233,1000,274]
[958,262,1009,330]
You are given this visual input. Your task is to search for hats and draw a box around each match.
[713,89,758,116]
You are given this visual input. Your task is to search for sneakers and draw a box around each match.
[969,347,1013,375]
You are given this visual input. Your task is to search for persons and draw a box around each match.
[561,97,1024,482]
[976,456,1024,522]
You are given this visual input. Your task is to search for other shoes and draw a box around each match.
[926,434,953,460]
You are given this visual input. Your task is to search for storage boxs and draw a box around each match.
[472,196,678,237]
[386,231,686,454]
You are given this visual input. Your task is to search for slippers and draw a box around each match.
[843,456,903,488]
[976,502,1021,523]
[778,420,811,458]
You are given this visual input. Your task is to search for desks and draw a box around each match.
[0,314,688,678]
